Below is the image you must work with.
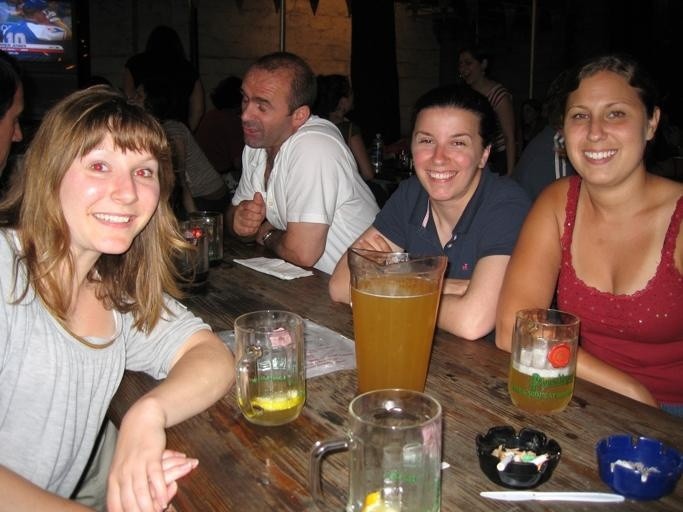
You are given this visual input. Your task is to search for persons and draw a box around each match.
[1,24,680,221]
[494,52,682,417]
[0,83,237,512]
[1,0,74,62]
[325,86,533,342]
[224,49,383,276]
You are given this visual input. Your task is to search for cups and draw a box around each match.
[508,308,581,418]
[369,134,383,167]
[189,210,223,262]
[309,388,442,511]
[172,221,209,280]
[346,245,448,402]
[231,309,307,427]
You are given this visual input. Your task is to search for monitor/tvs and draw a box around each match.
[0,0,90,72]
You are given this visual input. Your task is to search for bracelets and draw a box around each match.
[261,228,275,245]
